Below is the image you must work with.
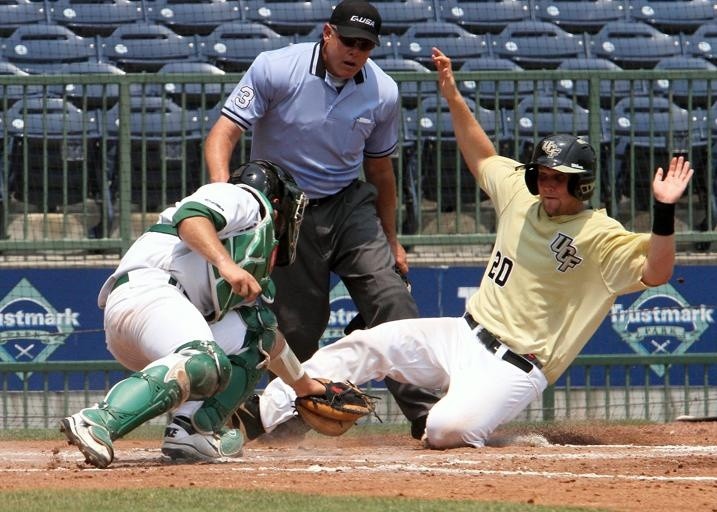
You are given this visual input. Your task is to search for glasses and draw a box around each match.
[331,26,375,50]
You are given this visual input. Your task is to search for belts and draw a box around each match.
[463,311,533,372]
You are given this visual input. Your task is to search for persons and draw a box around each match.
[57,158,384,471]
[202,1,445,447]
[223,45,695,451]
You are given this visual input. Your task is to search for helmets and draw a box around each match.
[228,159,308,267]
[516,135,596,201]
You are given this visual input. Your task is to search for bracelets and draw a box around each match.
[653,196,674,238]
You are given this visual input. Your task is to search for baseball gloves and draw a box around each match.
[294,377,373,436]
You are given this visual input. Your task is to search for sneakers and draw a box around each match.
[58,412,112,467]
[160,417,244,465]
[410,417,427,439]
[227,395,264,446]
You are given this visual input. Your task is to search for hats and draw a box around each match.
[329,0,382,46]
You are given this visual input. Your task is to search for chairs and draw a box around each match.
[1,1,717,242]
